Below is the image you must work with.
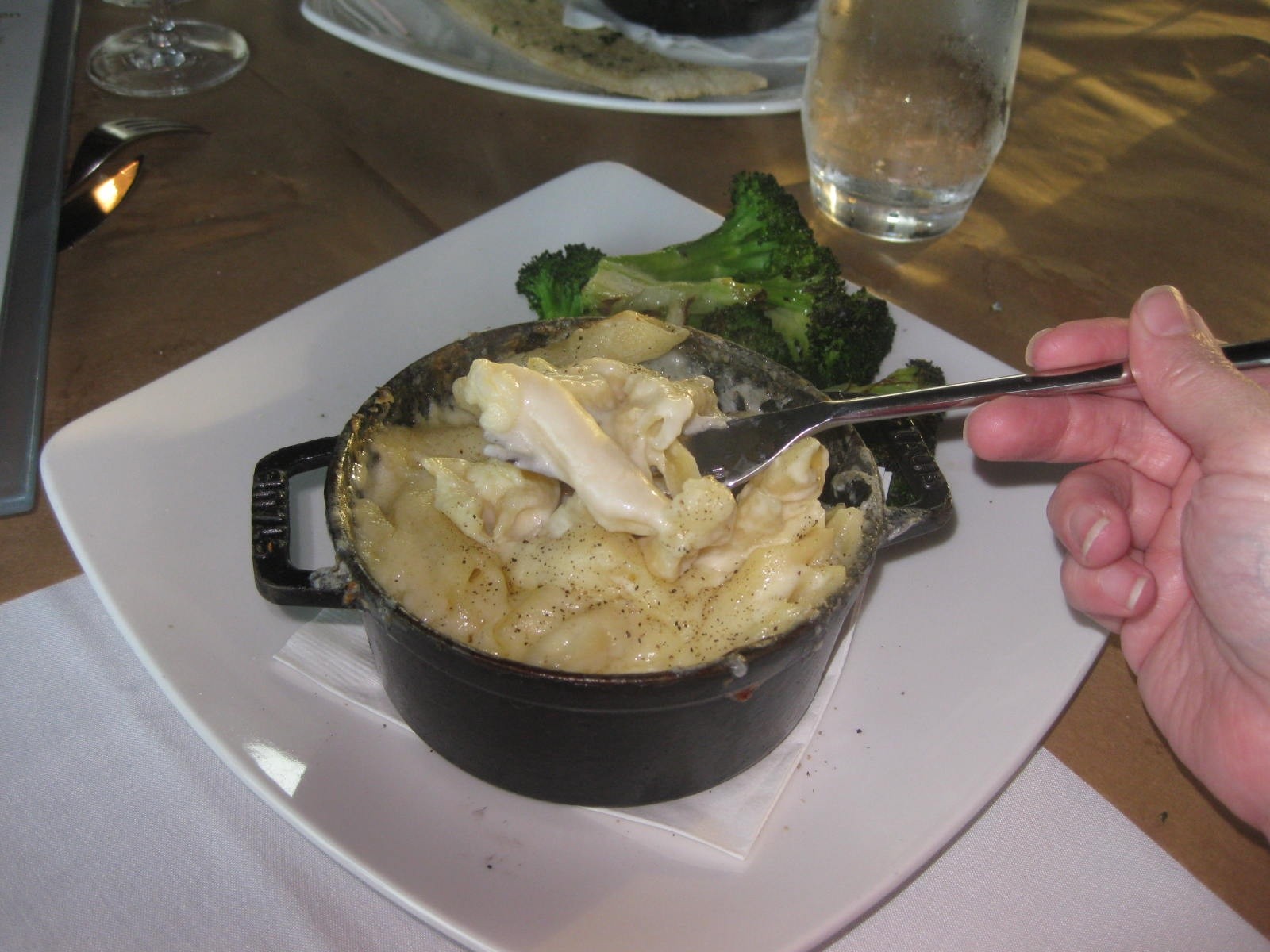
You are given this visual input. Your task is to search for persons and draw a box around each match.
[960,286,1270,842]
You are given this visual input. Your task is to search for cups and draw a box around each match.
[799,0,1028,244]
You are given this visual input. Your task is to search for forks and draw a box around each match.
[63,117,206,198]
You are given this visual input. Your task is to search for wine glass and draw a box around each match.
[84,0,251,100]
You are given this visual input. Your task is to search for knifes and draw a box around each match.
[57,159,140,253]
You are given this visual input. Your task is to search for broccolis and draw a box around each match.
[517,168,954,470]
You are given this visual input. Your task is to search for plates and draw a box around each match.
[36,157,1111,952]
[297,0,813,117]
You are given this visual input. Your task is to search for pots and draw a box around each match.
[249,314,958,810]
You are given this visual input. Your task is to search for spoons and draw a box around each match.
[650,333,1270,490]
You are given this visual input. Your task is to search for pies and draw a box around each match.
[445,1,768,101]
